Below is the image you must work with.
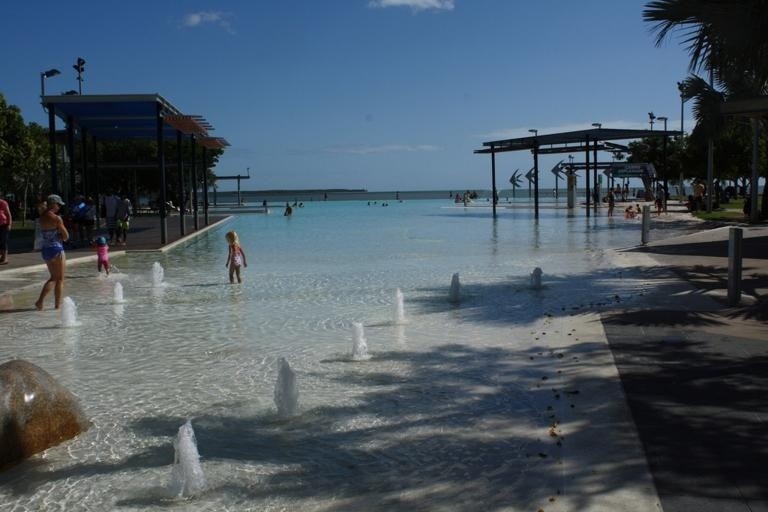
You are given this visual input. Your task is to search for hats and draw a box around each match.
[47,193,66,207]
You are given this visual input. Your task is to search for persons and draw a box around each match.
[34,194,69,313]
[592,180,667,220]
[0,197,14,264]
[447,189,478,207]
[684,183,707,212]
[283,200,304,217]
[90,236,111,275]
[224,230,248,283]
[62,185,134,250]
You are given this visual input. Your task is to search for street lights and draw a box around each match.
[648,111,655,130]
[527,129,538,137]
[41,69,60,97]
[593,122,601,130]
[72,56,86,95]
[657,115,669,213]
